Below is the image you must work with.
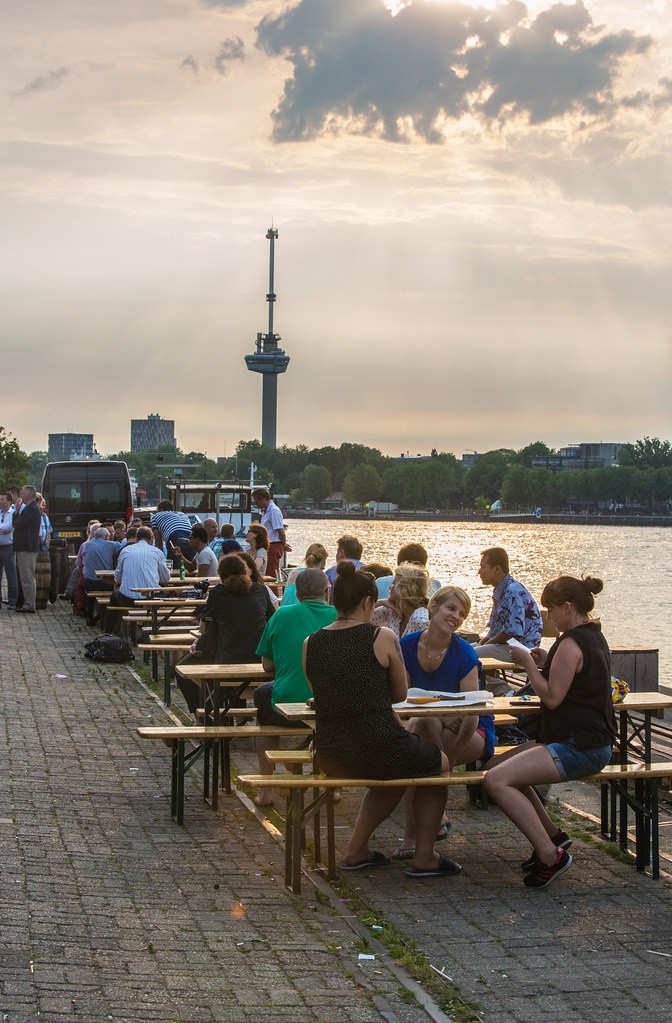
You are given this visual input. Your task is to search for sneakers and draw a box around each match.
[521,828,573,871]
[522,845,572,889]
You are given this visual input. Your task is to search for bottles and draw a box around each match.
[179,560,185,580]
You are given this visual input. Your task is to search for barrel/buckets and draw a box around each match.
[19,552,51,609]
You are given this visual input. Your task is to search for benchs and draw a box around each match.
[80,591,672,894]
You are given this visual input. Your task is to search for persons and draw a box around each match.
[254,568,344,806]
[393,586,494,859]
[0,483,440,635]
[467,547,543,698]
[12,485,41,613]
[301,561,463,878]
[483,573,618,889]
[174,555,276,726]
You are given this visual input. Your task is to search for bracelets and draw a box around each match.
[177,553,181,559]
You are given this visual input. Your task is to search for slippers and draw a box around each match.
[435,821,451,841]
[405,854,463,878]
[390,846,416,860]
[339,850,391,870]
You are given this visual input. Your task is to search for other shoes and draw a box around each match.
[7,604,16,610]
[15,607,36,613]
[59,594,71,600]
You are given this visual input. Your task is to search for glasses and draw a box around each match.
[187,536,195,541]
[1,515,4,523]
[248,530,254,534]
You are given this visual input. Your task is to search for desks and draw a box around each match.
[273,689,670,877]
[67,542,516,793]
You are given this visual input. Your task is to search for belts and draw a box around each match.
[173,540,187,544]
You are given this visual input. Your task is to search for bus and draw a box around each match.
[42,460,134,554]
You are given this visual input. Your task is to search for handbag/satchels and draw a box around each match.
[84,634,135,664]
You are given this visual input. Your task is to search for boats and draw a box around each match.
[135,483,289,540]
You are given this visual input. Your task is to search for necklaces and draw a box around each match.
[425,632,447,661]
[336,617,359,621]
